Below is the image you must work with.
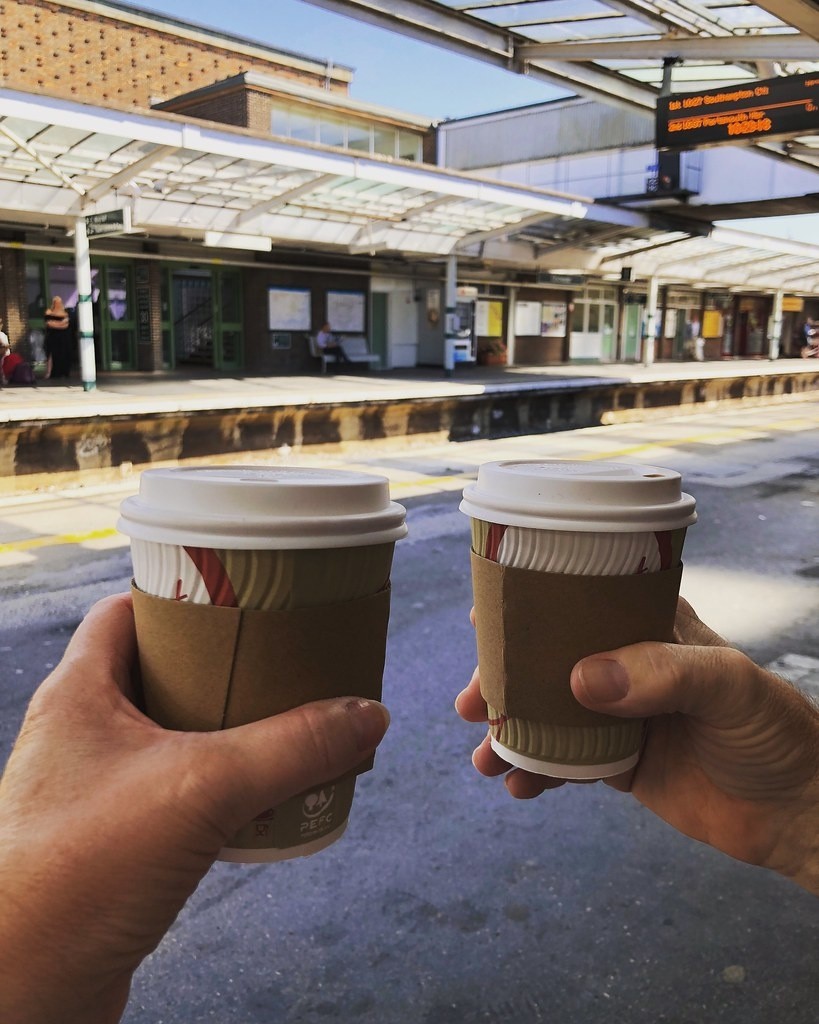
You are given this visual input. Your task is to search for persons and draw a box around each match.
[318,323,353,372]
[687,316,700,354]
[0,319,10,388]
[803,317,813,346]
[44,296,70,380]
[1,591,819,1024]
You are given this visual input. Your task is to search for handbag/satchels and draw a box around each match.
[11,362,34,383]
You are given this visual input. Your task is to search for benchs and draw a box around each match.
[308,336,379,376]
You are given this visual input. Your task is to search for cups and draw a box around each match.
[458,457,698,781]
[117,464,410,865]
